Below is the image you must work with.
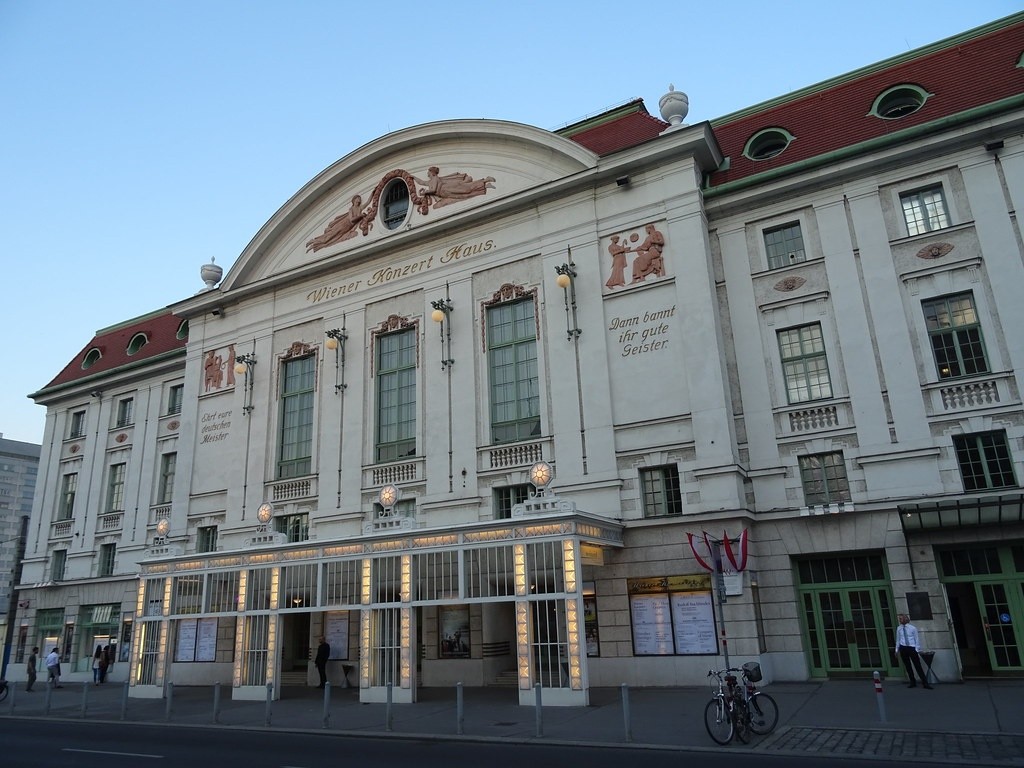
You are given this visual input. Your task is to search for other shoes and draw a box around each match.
[907,684,917,688]
[25,689,34,694]
[95,684,98,686]
[316,685,324,689]
[923,684,934,690]
[56,685,64,689]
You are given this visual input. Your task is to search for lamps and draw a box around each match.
[18,602,28,609]
[553,262,582,343]
[212,307,224,316]
[91,391,100,397]
[616,175,631,186]
[324,324,349,397]
[235,352,258,417]
[430,299,455,371]
[293,598,303,603]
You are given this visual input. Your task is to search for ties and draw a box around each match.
[903,626,909,645]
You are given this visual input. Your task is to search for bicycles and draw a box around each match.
[0,680,9,702]
[705,663,779,746]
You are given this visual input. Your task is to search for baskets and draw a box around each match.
[742,661,762,683]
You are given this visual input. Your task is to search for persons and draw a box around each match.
[785,278,795,291]
[388,314,398,328]
[92,645,109,686]
[71,445,76,452]
[408,167,496,199]
[305,189,375,253]
[47,647,64,689]
[501,283,513,298]
[205,344,236,390]
[929,246,941,257]
[119,434,125,442]
[605,224,665,290]
[894,614,934,689]
[315,635,330,689]
[170,421,177,429]
[292,341,302,355]
[25,647,39,692]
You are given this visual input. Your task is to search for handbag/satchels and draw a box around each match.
[99,660,106,669]
